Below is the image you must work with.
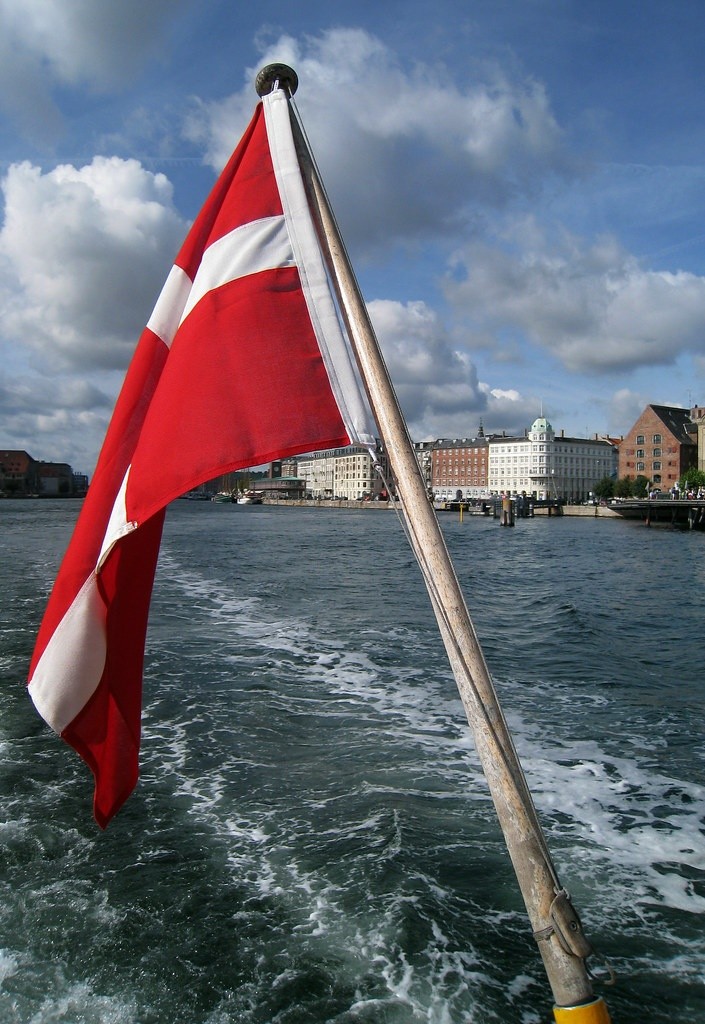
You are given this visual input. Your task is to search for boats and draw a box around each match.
[237,496,261,505]
[606,495,705,520]
[213,493,234,503]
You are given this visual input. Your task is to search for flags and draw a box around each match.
[26,82,379,830]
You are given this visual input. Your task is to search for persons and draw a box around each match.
[650,485,705,500]
